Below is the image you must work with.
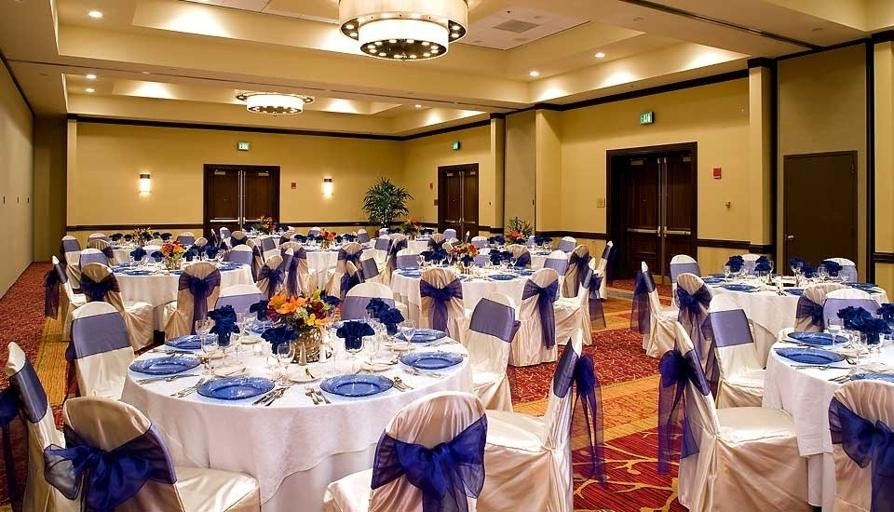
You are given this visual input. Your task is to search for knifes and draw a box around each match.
[253,387,289,407]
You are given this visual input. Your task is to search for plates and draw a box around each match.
[849,279,879,289]
[720,282,757,293]
[701,275,728,284]
[228,262,243,266]
[399,352,466,369]
[197,376,277,401]
[166,335,213,350]
[401,269,426,279]
[402,265,419,271]
[113,266,135,273]
[490,274,517,281]
[788,331,849,347]
[398,326,448,343]
[127,271,151,276]
[243,335,260,342]
[517,269,535,275]
[491,272,520,282]
[130,358,202,374]
[322,373,393,398]
[776,347,845,367]
[219,264,239,271]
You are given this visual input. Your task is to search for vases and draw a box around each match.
[461,264,469,273]
[165,258,182,270]
[409,233,416,240]
[320,240,330,249]
[259,230,269,235]
[289,327,322,362]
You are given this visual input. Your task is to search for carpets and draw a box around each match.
[20,282,80,512]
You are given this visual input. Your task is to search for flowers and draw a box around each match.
[400,217,421,235]
[452,243,478,269]
[505,216,535,246]
[253,216,273,235]
[160,240,187,258]
[132,226,154,240]
[321,229,336,241]
[264,288,335,332]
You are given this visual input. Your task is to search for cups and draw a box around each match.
[277,344,295,388]
[446,252,452,268]
[203,336,219,380]
[327,336,345,381]
[230,327,247,365]
[722,265,733,280]
[141,256,151,272]
[362,336,381,382]
[416,254,424,274]
[236,313,244,336]
[193,255,201,272]
[399,322,417,362]
[218,252,225,266]
[849,332,867,369]
[791,257,807,286]
[196,320,212,356]
[128,256,136,272]
[816,263,830,286]
[543,242,550,256]
[827,319,845,347]
[370,319,383,352]
[465,259,475,282]
[501,260,511,273]
[482,259,491,280]
[406,231,412,248]
[310,231,318,246]
[510,258,518,275]
[244,312,258,343]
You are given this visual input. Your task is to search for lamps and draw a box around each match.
[322,179,334,198]
[140,173,152,197]
[233,88,316,116]
[338,0,470,62]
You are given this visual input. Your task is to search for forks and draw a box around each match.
[170,377,209,398]
[828,370,862,385]
[392,379,414,393]
[311,388,323,402]
[403,363,446,380]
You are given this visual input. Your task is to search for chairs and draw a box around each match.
[61,234,82,289]
[80,248,108,271]
[307,226,320,237]
[471,236,488,250]
[558,236,577,253]
[341,282,395,320]
[358,229,370,243]
[177,232,195,245]
[396,248,419,268]
[701,309,766,409]
[279,232,291,245]
[89,233,106,241]
[79,263,153,353]
[5,342,82,512]
[323,391,489,512]
[281,242,319,298]
[673,273,713,373]
[821,258,857,283]
[630,261,679,358]
[53,255,88,341]
[508,268,559,368]
[822,289,884,329]
[590,240,614,300]
[228,244,253,264]
[657,320,809,512]
[87,239,115,266]
[671,253,701,310]
[44,396,259,512]
[360,248,382,283]
[340,260,362,298]
[385,233,407,270]
[245,241,265,281]
[557,245,592,298]
[464,230,470,243]
[214,285,268,327]
[443,229,457,241]
[260,236,278,262]
[379,227,388,236]
[419,268,473,348]
[828,380,894,512]
[777,283,852,338]
[724,253,769,278]
[462,292,521,413]
[64,300,135,402]
[373,234,392,274]
[325,242,366,300]
[551,257,606,346]
[156,261,221,342]
[231,231,247,247]
[477,329,609,512]
[506,244,531,267]
[219,227,233,250]
[255,255,285,300]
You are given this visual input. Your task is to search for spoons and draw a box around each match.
[305,392,319,405]
[316,390,331,404]
